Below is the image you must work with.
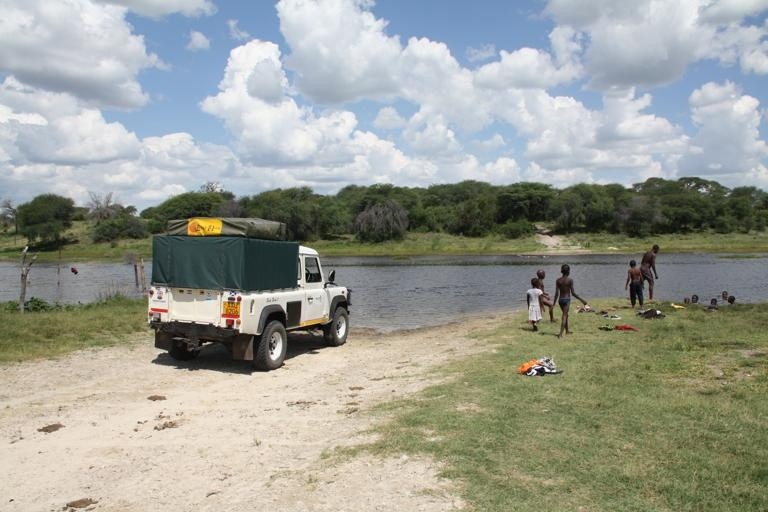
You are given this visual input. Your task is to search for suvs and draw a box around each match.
[149,217,353,371]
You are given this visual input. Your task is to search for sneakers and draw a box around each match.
[596,311,621,320]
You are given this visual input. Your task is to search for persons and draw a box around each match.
[625,259,645,310]
[692,295,699,303]
[552,264,588,338]
[640,245,659,300]
[536,269,559,323]
[526,278,546,331]
[722,291,728,300]
[710,298,717,305]
[728,295,736,305]
[684,296,691,304]
[71,265,79,275]
